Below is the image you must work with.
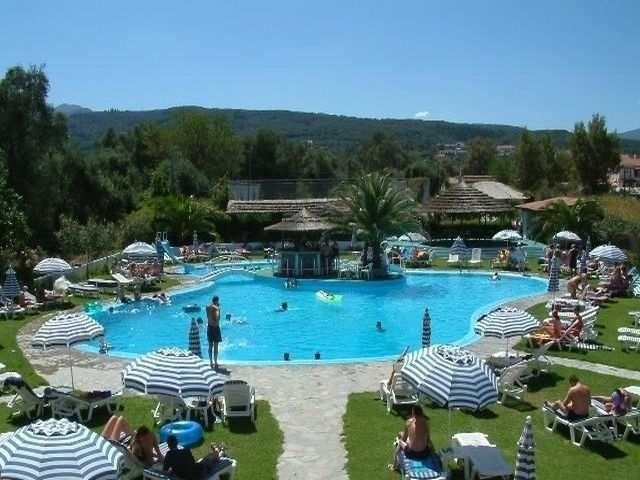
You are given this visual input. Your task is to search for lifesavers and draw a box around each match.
[84,300,102,310]
[160,420,203,445]
[183,304,201,313]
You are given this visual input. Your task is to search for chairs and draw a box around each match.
[379,246,640,480]
[0,245,256,480]
[336,260,374,280]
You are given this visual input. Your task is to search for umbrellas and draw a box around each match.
[553,230,582,244]
[0,416,128,480]
[492,228,522,248]
[513,415,537,480]
[118,345,225,403]
[122,239,157,256]
[397,232,429,243]
[33,254,73,274]
[449,235,470,270]
[32,309,105,392]
[401,343,498,440]
[420,307,432,347]
[472,304,544,359]
[0,264,21,312]
[188,317,202,358]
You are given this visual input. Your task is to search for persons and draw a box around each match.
[129,424,163,465]
[538,309,561,337]
[544,374,591,420]
[386,242,428,265]
[180,244,209,259]
[102,414,136,442]
[546,242,631,300]
[498,249,506,263]
[591,385,633,417]
[163,435,223,480]
[397,404,431,459]
[206,295,221,368]
[569,307,583,336]
